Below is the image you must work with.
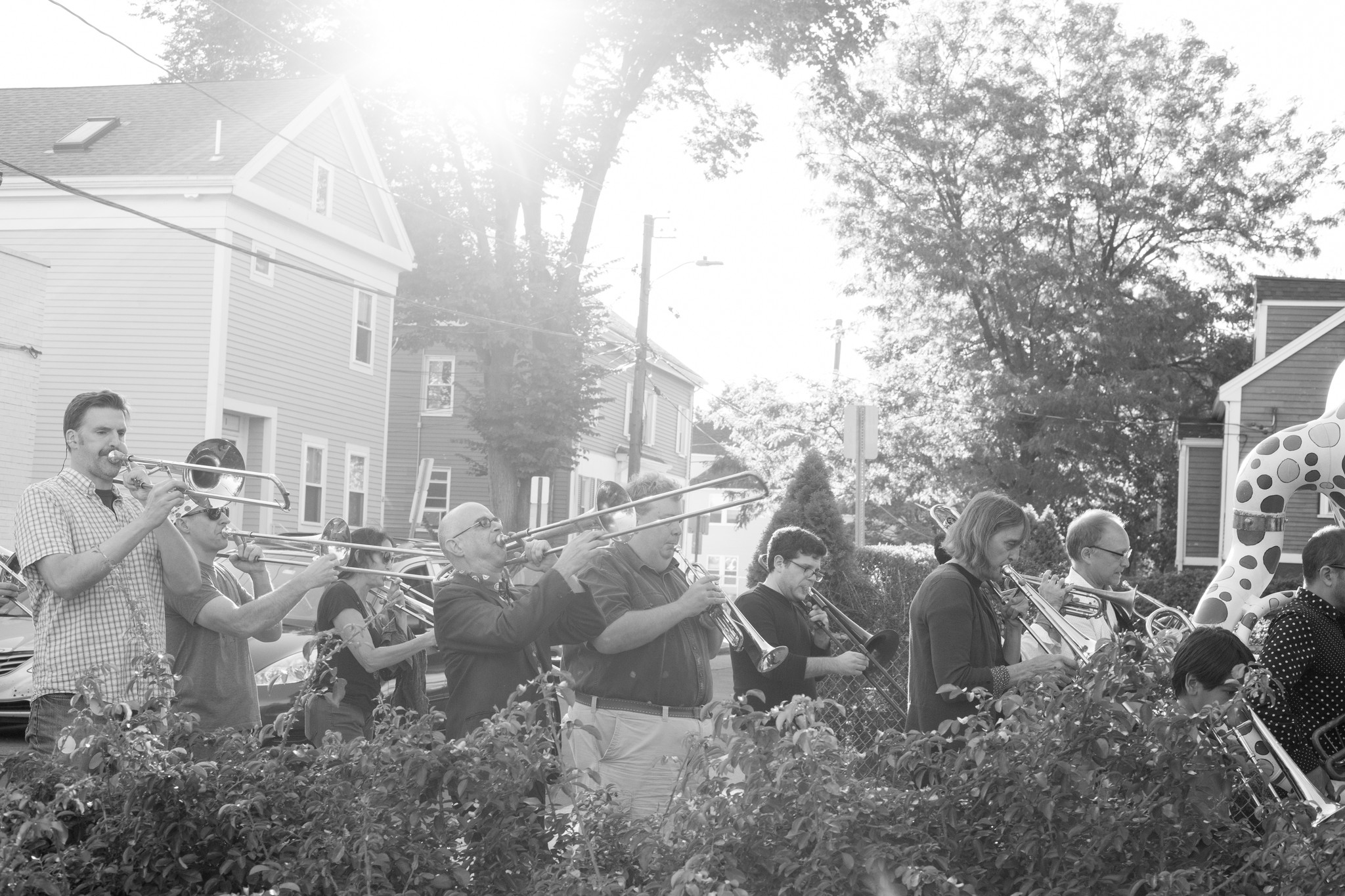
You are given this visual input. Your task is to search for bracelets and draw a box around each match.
[1034,620,1053,629]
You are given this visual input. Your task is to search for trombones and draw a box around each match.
[108,438,290,510]
[216,517,447,581]
[757,553,908,721]
[1146,606,1345,892]
[433,470,769,587]
[312,555,434,628]
[929,503,1145,730]
[0,545,33,617]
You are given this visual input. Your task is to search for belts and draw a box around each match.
[575,693,713,720]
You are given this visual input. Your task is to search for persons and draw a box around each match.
[1246,526,1345,896]
[13,390,188,769]
[935,529,1076,662]
[1169,627,1256,852]
[561,472,731,871]
[1062,508,1174,674]
[163,495,342,762]
[433,502,607,869]
[303,529,437,748]
[730,525,869,774]
[902,491,1080,791]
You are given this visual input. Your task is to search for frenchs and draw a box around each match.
[1188,359,1344,807]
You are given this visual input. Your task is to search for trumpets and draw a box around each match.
[1120,580,1193,632]
[1002,572,1139,620]
[673,544,788,673]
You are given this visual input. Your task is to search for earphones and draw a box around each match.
[71,438,76,443]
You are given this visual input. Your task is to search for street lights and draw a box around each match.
[627,214,725,486]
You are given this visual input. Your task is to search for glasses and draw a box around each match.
[783,557,825,582]
[377,551,394,565]
[1089,546,1132,559]
[181,507,229,520]
[452,518,503,538]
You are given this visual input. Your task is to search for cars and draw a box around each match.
[0,589,43,735]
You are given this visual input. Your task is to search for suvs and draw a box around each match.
[203,532,450,746]
